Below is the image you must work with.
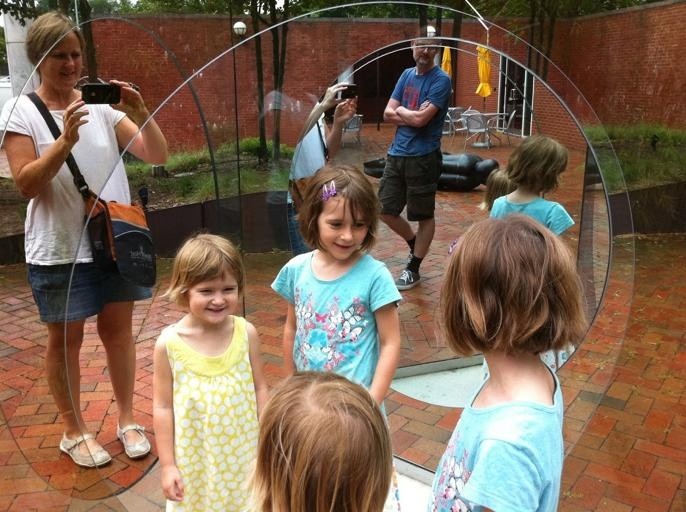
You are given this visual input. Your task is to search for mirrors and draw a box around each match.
[282,39,615,415]
[2,0,615,512]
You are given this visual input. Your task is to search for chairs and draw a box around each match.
[441,104,517,153]
[341,112,364,149]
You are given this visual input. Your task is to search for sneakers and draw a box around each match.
[59,430,112,467]
[395,268,421,290]
[407,252,414,267]
[116,420,151,458]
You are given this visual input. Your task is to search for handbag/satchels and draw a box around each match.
[292,178,314,214]
[82,189,156,288]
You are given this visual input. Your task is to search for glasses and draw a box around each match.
[414,40,440,49]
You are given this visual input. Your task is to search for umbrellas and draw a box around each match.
[440,46,456,107]
[474,44,495,140]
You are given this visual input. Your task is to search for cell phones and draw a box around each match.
[334,85,358,99]
[81,83,121,104]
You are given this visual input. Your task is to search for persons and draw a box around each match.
[266,157,401,413]
[151,232,268,512]
[377,38,455,291]
[485,134,573,376]
[485,168,519,206]
[2,6,170,469]
[256,46,358,267]
[426,213,590,512]
[244,371,392,512]
